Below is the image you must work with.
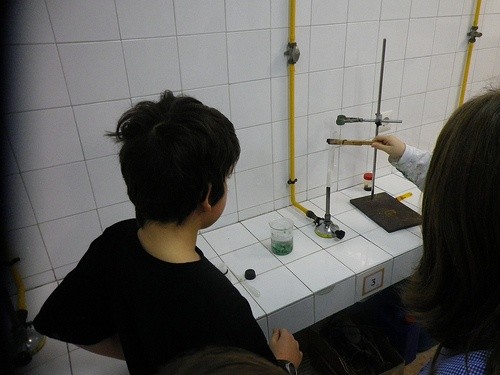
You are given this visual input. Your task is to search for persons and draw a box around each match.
[33,90,305,375]
[154,346,290,375]
[372,88,500,375]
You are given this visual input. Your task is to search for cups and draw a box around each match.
[269,217,294,256]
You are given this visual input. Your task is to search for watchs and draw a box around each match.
[277,360,297,375]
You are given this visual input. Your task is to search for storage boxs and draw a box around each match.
[305,307,441,375]
